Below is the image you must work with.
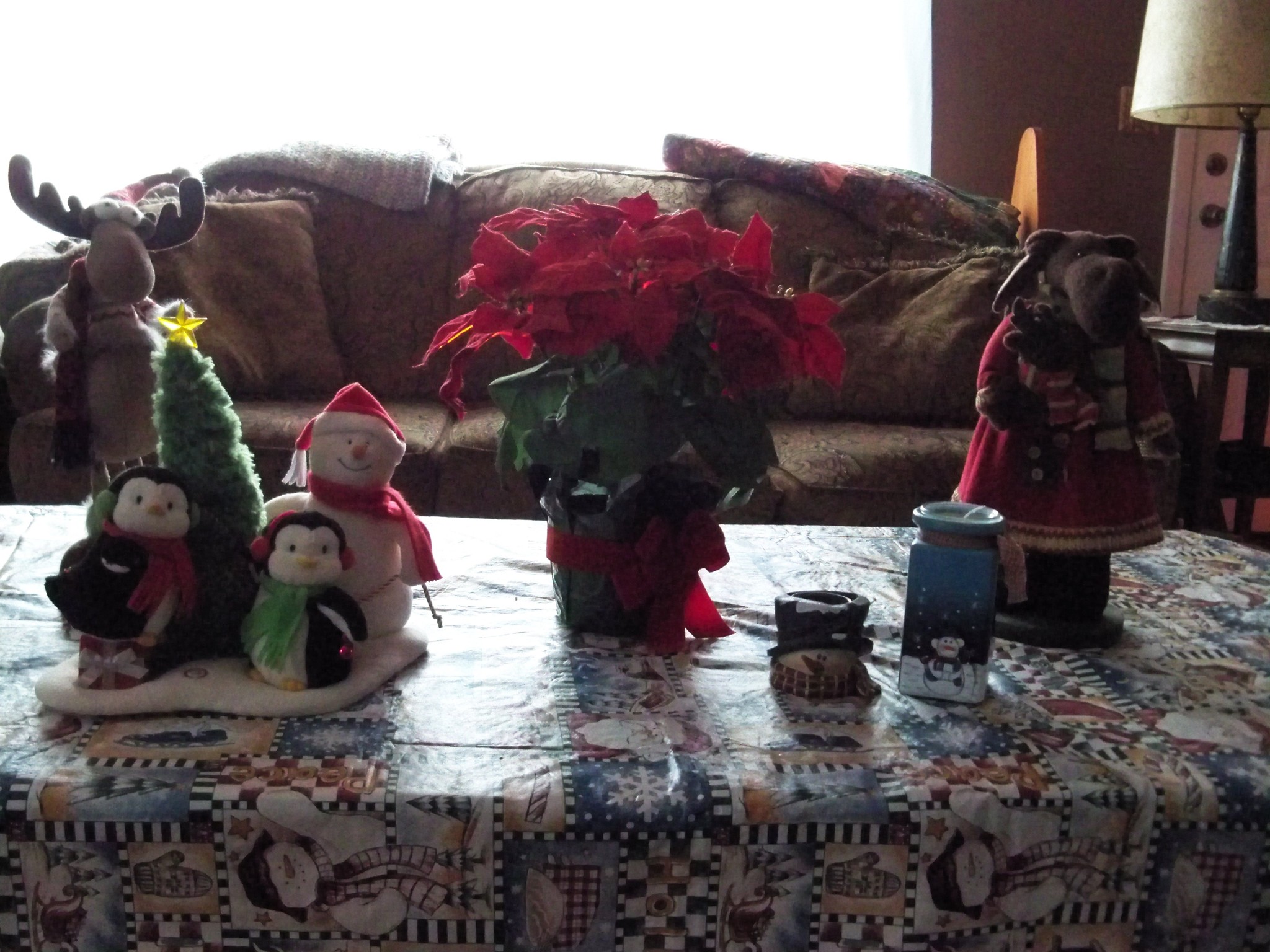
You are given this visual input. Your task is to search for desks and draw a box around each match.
[1143,317,1270,540]
[1,502,1270,952]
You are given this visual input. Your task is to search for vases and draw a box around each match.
[539,475,700,644]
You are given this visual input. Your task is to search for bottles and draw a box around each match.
[898,501,1005,704]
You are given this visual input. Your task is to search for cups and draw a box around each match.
[767,589,881,700]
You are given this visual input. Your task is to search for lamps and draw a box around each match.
[1131,0,1270,325]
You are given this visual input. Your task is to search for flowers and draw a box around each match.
[412,192,850,519]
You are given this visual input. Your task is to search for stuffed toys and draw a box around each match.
[263,384,442,638]
[46,467,196,661]
[3,156,204,505]
[243,512,369,691]
[953,229,1174,619]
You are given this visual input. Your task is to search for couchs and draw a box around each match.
[0,133,1183,524]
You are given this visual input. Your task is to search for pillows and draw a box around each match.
[799,222,1024,428]
[123,189,347,396]
